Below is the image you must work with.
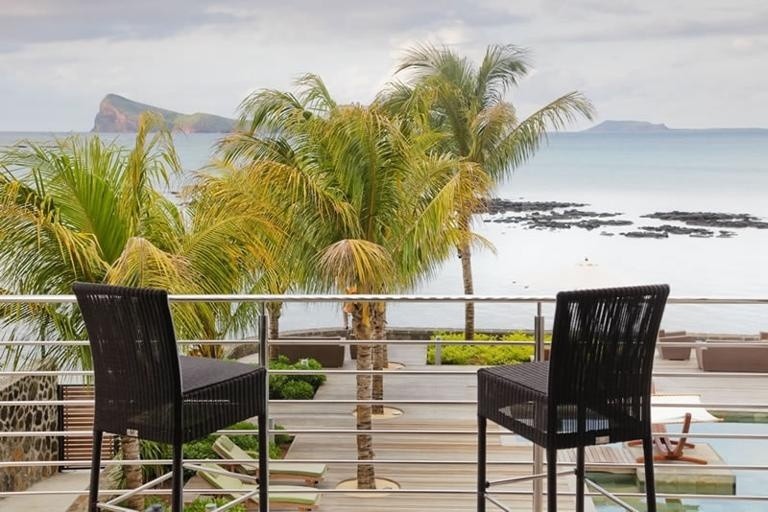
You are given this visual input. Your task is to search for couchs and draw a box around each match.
[696,340,768,373]
[269,336,344,367]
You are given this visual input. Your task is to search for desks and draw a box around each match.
[627,394,724,463]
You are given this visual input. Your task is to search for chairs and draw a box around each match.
[350,333,357,359]
[196,458,320,512]
[71,281,266,512]
[745,333,768,340]
[211,434,327,487]
[657,330,691,360]
[477,284,670,512]
